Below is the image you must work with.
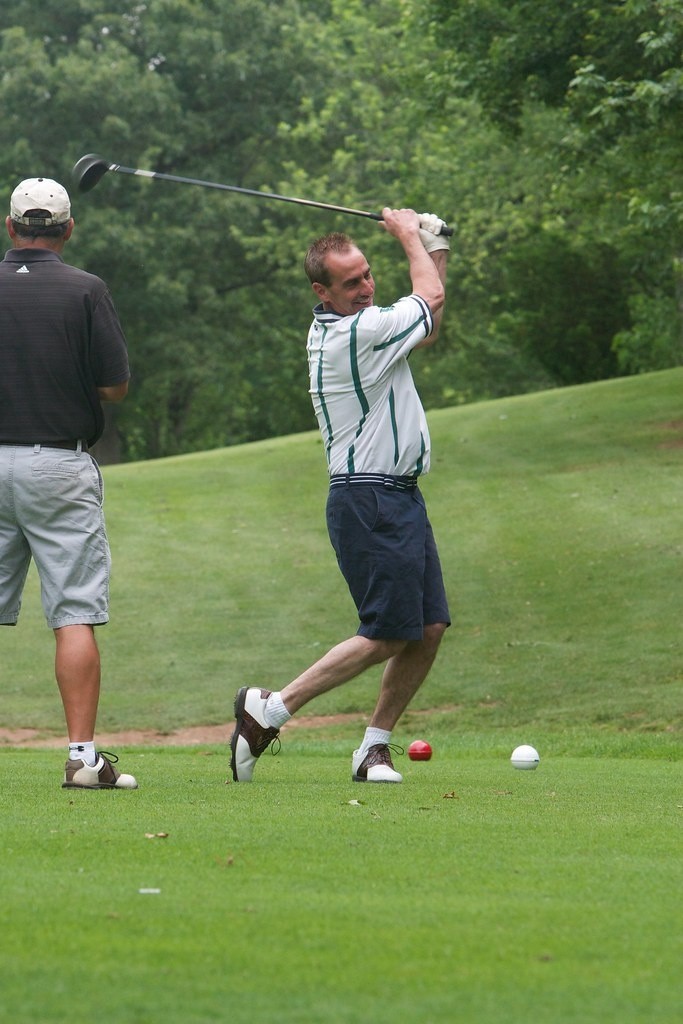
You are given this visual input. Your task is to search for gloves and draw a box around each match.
[416,213,451,254]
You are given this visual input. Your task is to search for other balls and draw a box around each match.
[408,739,432,761]
[510,744,541,770]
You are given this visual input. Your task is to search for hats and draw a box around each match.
[9,177,71,226]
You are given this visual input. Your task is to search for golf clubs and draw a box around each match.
[70,152,455,238]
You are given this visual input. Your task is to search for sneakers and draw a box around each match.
[61,750,139,790]
[350,742,405,783]
[227,686,281,783]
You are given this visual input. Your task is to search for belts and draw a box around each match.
[1,438,91,452]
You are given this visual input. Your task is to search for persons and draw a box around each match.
[1,178,137,788]
[230,207,449,783]
